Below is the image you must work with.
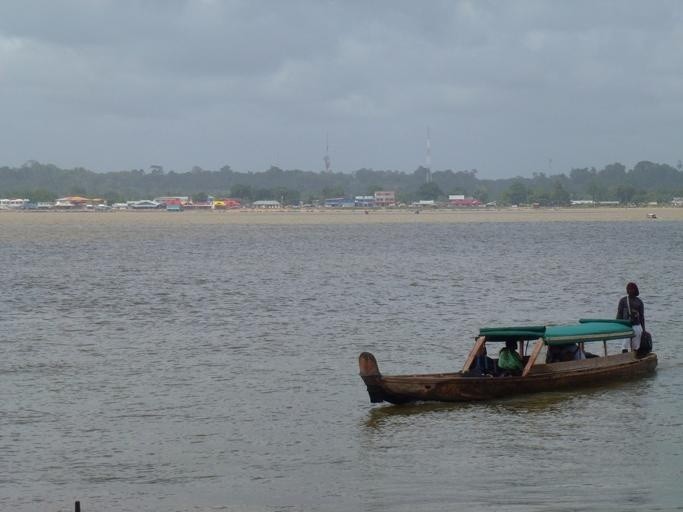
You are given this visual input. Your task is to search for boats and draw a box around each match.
[358,320,659,408]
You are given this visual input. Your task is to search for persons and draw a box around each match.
[615,283,646,354]
[498,339,525,371]
[545,342,586,364]
[469,343,501,379]
